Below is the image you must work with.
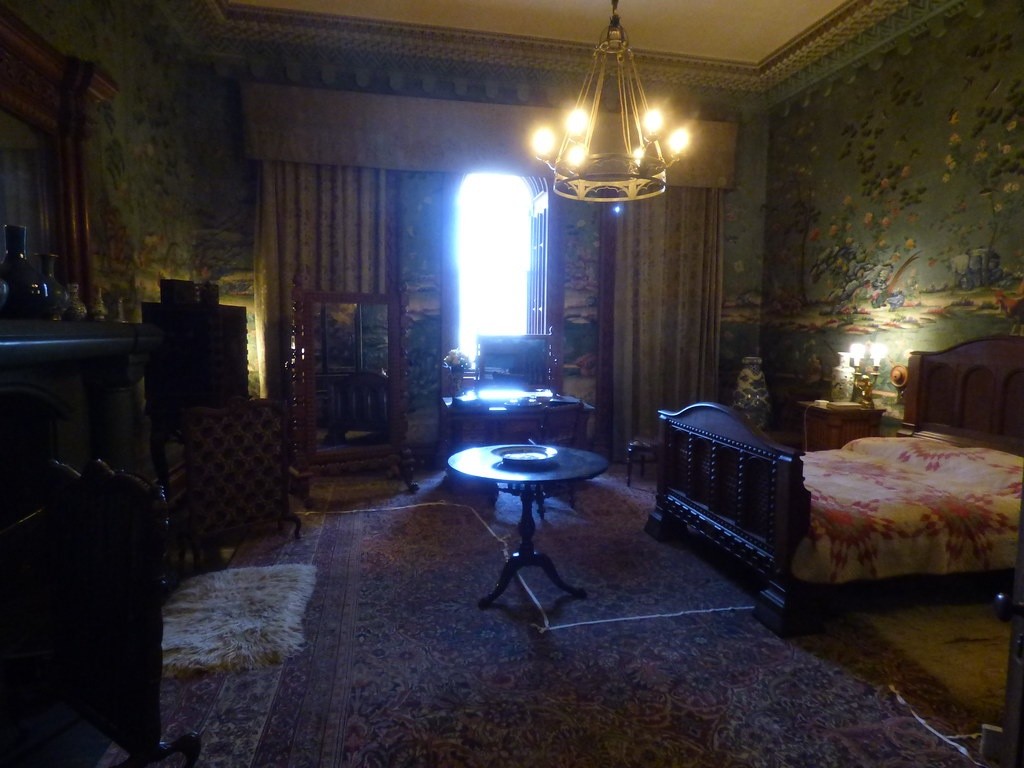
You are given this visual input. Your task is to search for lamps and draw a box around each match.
[532,0,691,201]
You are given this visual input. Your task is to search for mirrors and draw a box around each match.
[288,292,418,508]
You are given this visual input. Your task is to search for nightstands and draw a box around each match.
[797,400,887,450]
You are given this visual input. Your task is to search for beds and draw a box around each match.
[643,335,1024,627]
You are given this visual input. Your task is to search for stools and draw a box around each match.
[625,437,663,483]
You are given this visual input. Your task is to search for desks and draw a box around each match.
[445,442,605,607]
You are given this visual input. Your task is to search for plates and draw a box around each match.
[491,444,558,465]
